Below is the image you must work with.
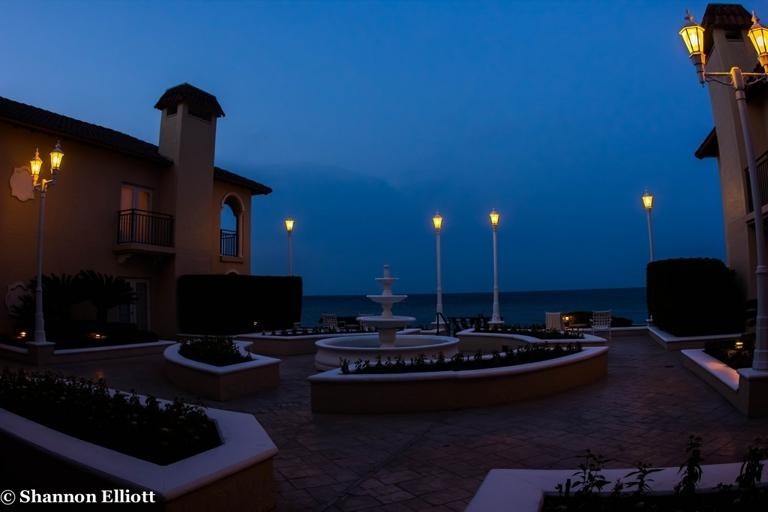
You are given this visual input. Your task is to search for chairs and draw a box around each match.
[588,308,613,341]
[320,310,346,332]
[545,311,562,333]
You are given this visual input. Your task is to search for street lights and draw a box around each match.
[284,212,295,274]
[432,207,449,326]
[27,139,63,347]
[640,188,656,261]
[488,205,504,327]
[677,4,767,372]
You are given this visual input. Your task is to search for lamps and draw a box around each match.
[560,310,575,328]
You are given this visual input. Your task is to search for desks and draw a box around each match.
[568,324,592,335]
[343,324,364,333]
